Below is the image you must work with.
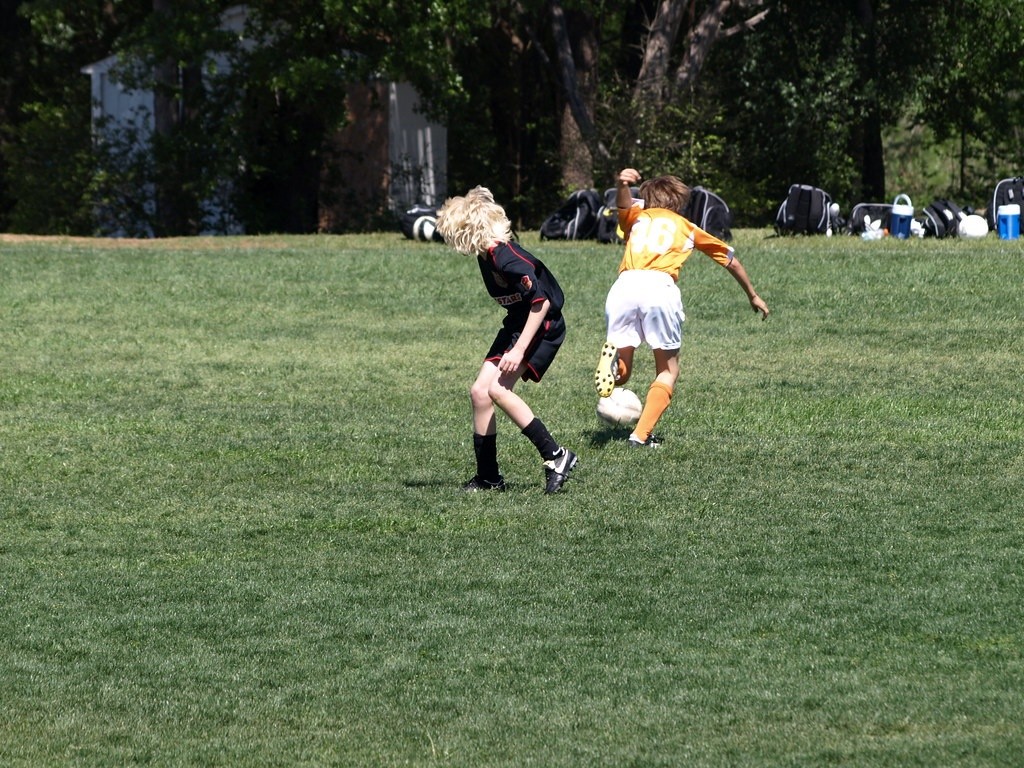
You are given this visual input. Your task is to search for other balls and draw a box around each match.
[957,214,989,241]
[412,215,439,244]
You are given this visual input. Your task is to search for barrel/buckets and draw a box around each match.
[996,203,1021,239]
[888,193,914,240]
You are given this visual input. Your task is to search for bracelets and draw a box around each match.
[751,294,759,300]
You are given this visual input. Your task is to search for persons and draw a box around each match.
[436,185,579,496]
[594,168,772,451]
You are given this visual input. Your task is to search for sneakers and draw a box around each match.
[461,475,506,492]
[628,432,660,447]
[543,446,579,495]
[594,342,620,398]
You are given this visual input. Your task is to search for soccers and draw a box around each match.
[593,387,642,426]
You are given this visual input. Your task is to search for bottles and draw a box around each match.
[860,229,888,240]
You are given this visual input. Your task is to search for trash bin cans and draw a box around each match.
[890,204,916,238]
[997,203,1020,240]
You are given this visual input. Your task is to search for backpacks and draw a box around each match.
[396,204,443,242]
[596,187,641,244]
[847,203,893,236]
[538,188,605,241]
[683,185,732,242]
[984,175,1024,234]
[773,184,845,237]
[920,199,974,240]
